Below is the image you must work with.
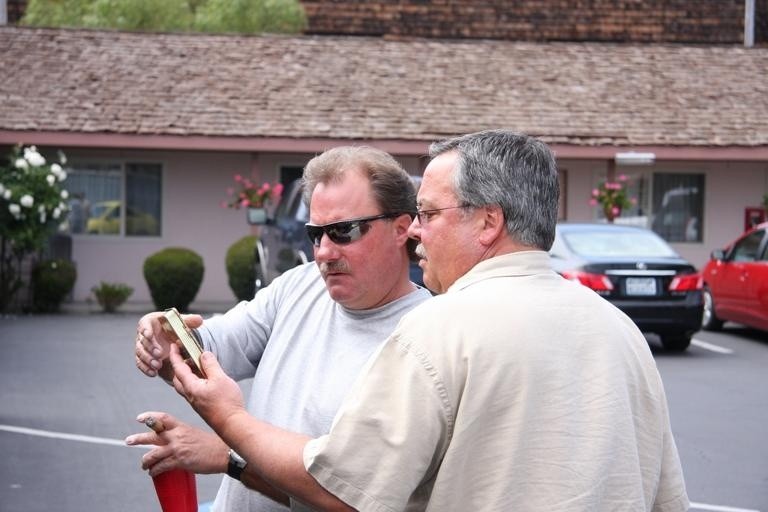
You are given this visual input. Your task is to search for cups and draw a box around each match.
[149,458,199,512]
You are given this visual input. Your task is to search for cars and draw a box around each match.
[669,221,768,343]
[243,173,452,307]
[552,222,706,356]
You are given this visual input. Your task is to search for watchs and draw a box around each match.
[225,445,248,481]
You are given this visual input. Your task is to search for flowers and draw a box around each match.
[585,173,640,222]
[0,140,73,261]
[220,173,286,211]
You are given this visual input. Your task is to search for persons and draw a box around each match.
[168,127,692,512]
[126,143,433,511]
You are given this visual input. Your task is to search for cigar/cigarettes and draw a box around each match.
[144,415,166,434]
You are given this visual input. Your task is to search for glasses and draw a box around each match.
[305,214,396,246]
[416,202,475,224]
